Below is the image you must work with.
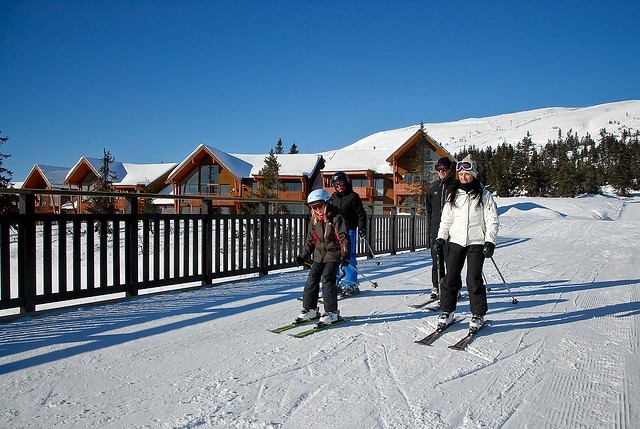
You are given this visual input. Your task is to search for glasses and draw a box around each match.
[456,161,473,171]
[311,202,325,210]
[435,164,449,171]
[332,179,348,187]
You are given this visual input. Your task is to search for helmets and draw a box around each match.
[331,173,350,187]
[307,189,330,206]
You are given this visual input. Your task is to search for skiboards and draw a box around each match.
[413,312,490,349]
[297,292,346,304]
[267,315,355,338]
[410,288,469,312]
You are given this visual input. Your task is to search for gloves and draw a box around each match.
[432,238,445,254]
[340,253,351,266]
[483,242,495,258]
[295,255,304,266]
[358,228,367,240]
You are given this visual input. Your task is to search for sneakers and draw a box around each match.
[342,284,359,296]
[296,307,319,322]
[429,287,439,299]
[336,284,342,294]
[319,310,340,325]
[437,311,455,326]
[469,313,484,331]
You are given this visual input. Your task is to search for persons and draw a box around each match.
[318,170,367,298]
[425,157,460,298]
[433,154,499,334]
[293,188,350,327]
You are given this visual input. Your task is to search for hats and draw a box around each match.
[437,157,451,169]
[456,154,479,179]
